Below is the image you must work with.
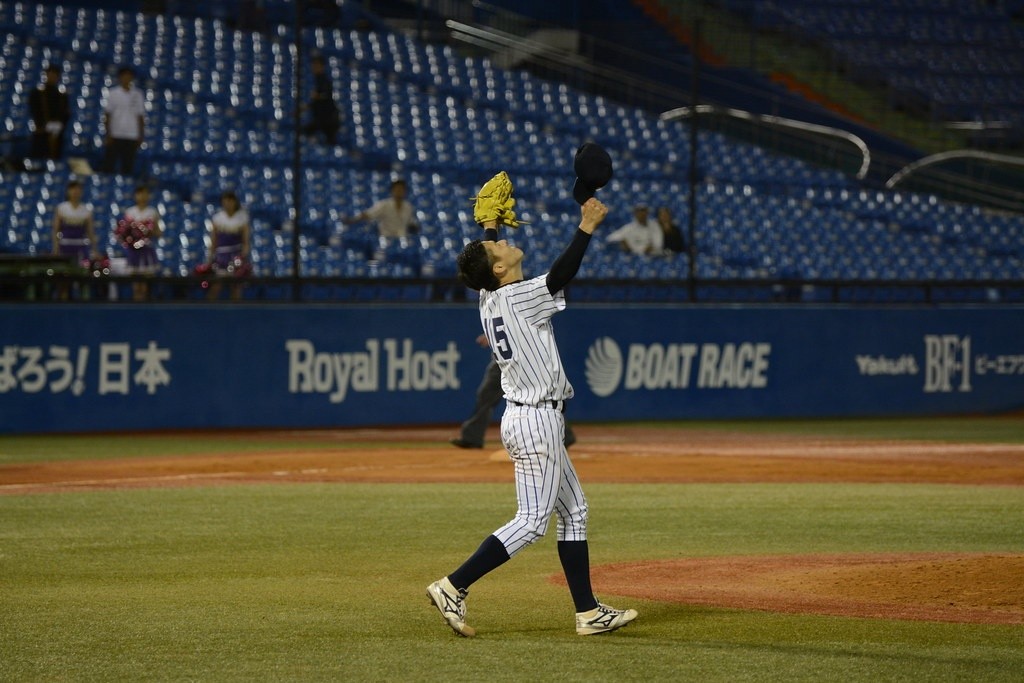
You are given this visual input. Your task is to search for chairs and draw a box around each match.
[0,0,1022,298]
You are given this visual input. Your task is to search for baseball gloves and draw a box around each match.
[472,169,520,230]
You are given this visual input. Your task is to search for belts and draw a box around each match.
[516,400,565,412]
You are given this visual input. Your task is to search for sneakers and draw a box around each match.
[426,581,475,637]
[575,597,637,636]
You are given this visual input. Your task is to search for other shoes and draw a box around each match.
[449,438,483,448]
[564,432,575,448]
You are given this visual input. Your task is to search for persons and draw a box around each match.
[425,171,637,635]
[122,185,165,303]
[339,180,421,239]
[297,53,341,144]
[20,63,72,173]
[447,332,576,451]
[604,203,687,253]
[98,66,146,175]
[48,178,98,301]
[192,192,251,299]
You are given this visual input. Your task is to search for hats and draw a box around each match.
[573,143,613,205]
